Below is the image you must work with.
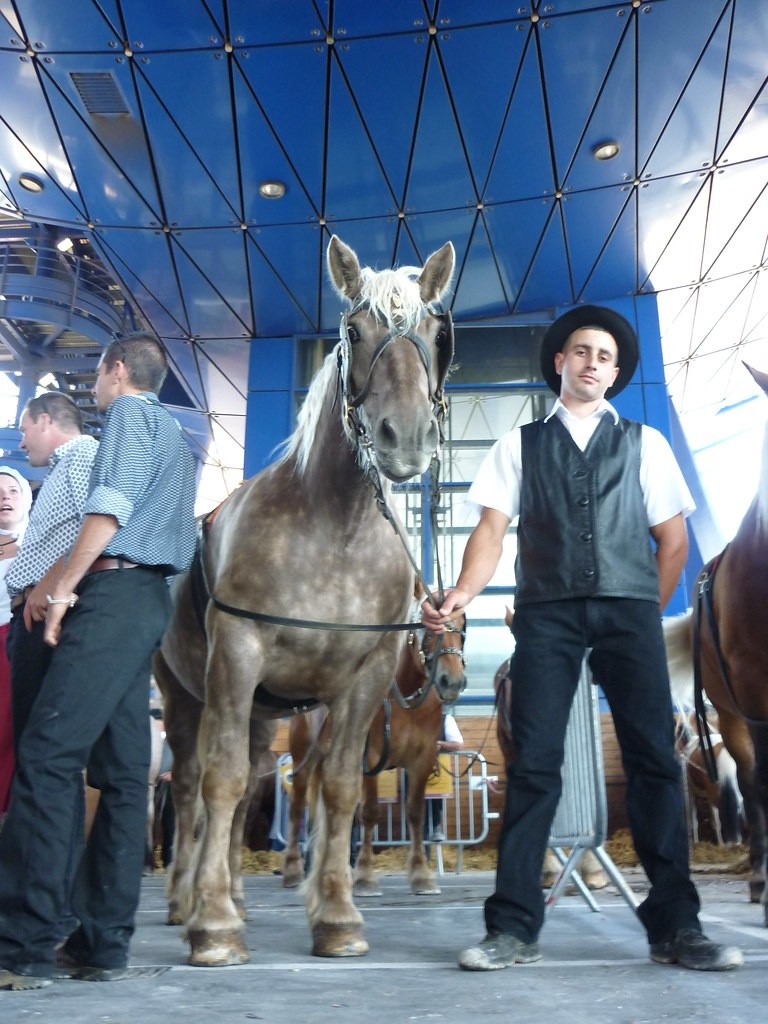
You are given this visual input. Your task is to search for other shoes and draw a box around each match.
[55,946,126,981]
[432,830,445,841]
[0,966,53,990]
[142,866,154,876]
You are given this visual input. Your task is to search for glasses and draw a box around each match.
[34,395,51,424]
[112,331,126,363]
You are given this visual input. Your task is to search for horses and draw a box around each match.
[661,359,767,931]
[280,572,468,898]
[156,232,457,970]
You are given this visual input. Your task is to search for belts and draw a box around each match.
[10,586,36,613]
[84,558,140,573]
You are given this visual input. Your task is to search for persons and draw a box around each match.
[420,304,745,970]
[424,712,463,859]
[0,335,197,992]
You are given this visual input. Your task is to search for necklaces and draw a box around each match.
[0,538,18,555]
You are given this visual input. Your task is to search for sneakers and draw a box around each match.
[650,926,743,970]
[458,930,542,970]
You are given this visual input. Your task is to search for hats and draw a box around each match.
[149,709,164,719]
[0,465,32,513]
[540,304,640,401]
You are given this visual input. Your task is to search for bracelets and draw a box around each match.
[47,592,78,607]
